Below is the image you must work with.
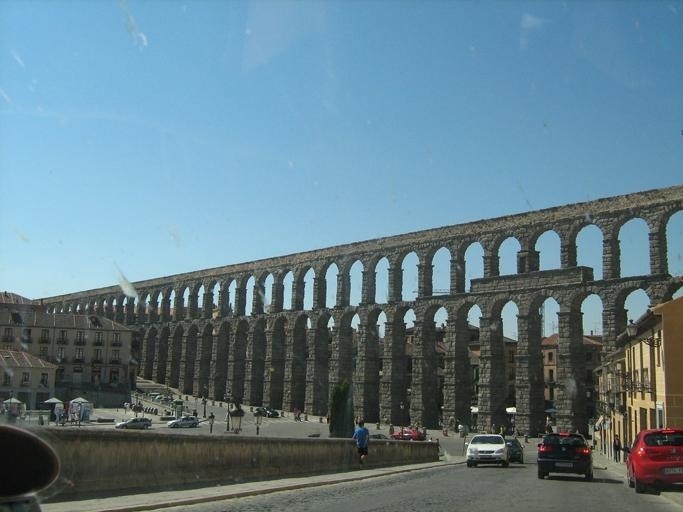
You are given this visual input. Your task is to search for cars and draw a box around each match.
[465,434,525,467]
[537,433,593,480]
[115,418,152,429]
[254,407,279,418]
[167,415,199,428]
[392,428,424,441]
[622,426,683,493]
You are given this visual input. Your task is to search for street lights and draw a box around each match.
[224,390,232,431]
[202,384,209,418]
[253,412,263,436]
[400,399,405,440]
[208,412,215,433]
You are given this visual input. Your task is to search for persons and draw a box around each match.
[458,423,463,435]
[352,419,370,470]
[409,437,413,440]
[613,435,621,464]
[428,437,432,441]
[491,424,496,434]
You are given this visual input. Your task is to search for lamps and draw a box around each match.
[624,318,661,349]
